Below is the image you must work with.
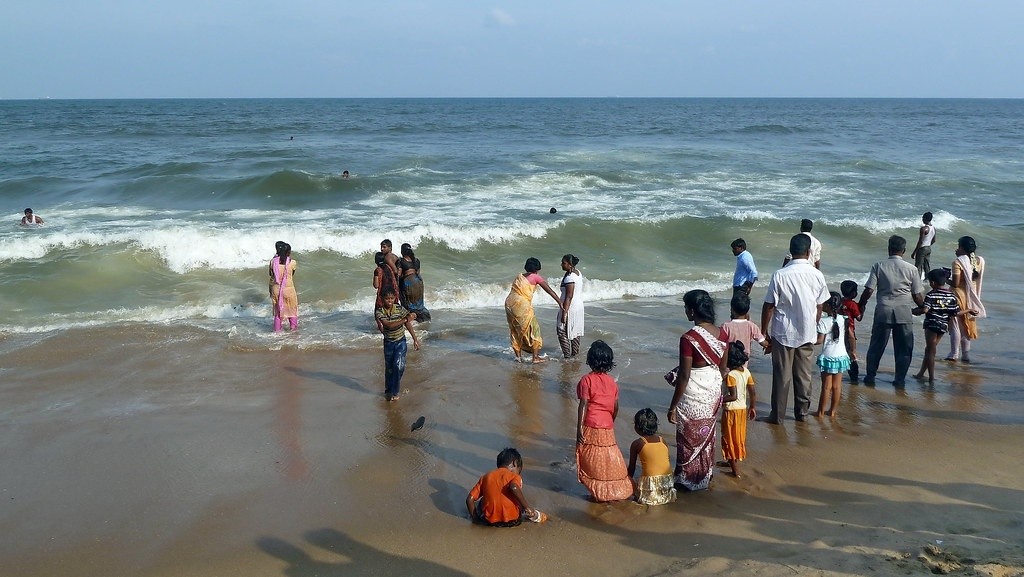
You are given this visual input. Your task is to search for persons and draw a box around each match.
[556,254,585,358]
[910,212,936,275]
[627,407,679,506]
[837,281,862,387]
[575,338,636,502]
[858,234,924,389]
[717,339,757,478]
[946,237,987,363]
[913,268,980,385]
[780,219,823,271]
[373,252,399,333]
[342,170,349,178]
[374,285,419,402]
[465,448,547,529]
[20,208,44,224]
[815,291,853,418]
[505,257,566,364]
[381,238,399,276]
[666,290,729,492]
[395,243,432,323]
[729,238,758,321]
[756,234,832,428]
[720,294,769,368]
[269,241,298,333]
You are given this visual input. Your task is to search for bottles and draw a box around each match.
[942,267,951,279]
[530,510,547,523]
